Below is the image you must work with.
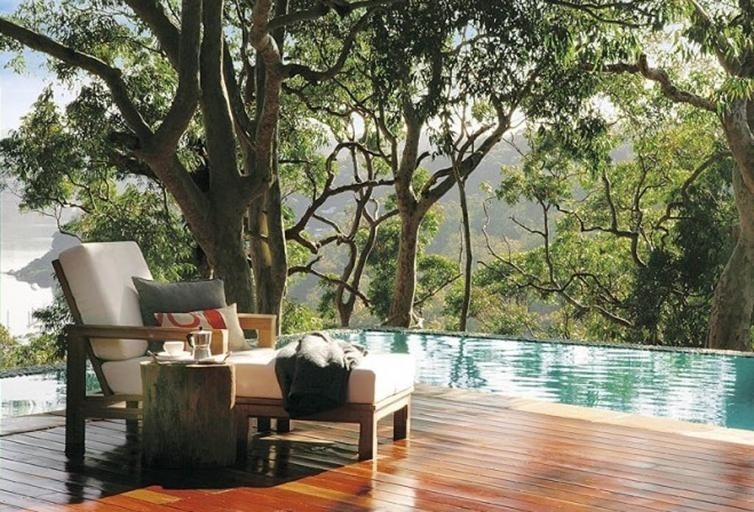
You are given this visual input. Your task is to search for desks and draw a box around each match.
[136,358,237,489]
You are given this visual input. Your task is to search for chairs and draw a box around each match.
[47,240,416,470]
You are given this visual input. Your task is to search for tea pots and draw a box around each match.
[187,326,212,360]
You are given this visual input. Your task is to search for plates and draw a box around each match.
[154,352,190,360]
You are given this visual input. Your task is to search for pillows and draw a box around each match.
[128,274,226,356]
[150,300,251,357]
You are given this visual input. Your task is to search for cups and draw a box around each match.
[164,340,182,354]
